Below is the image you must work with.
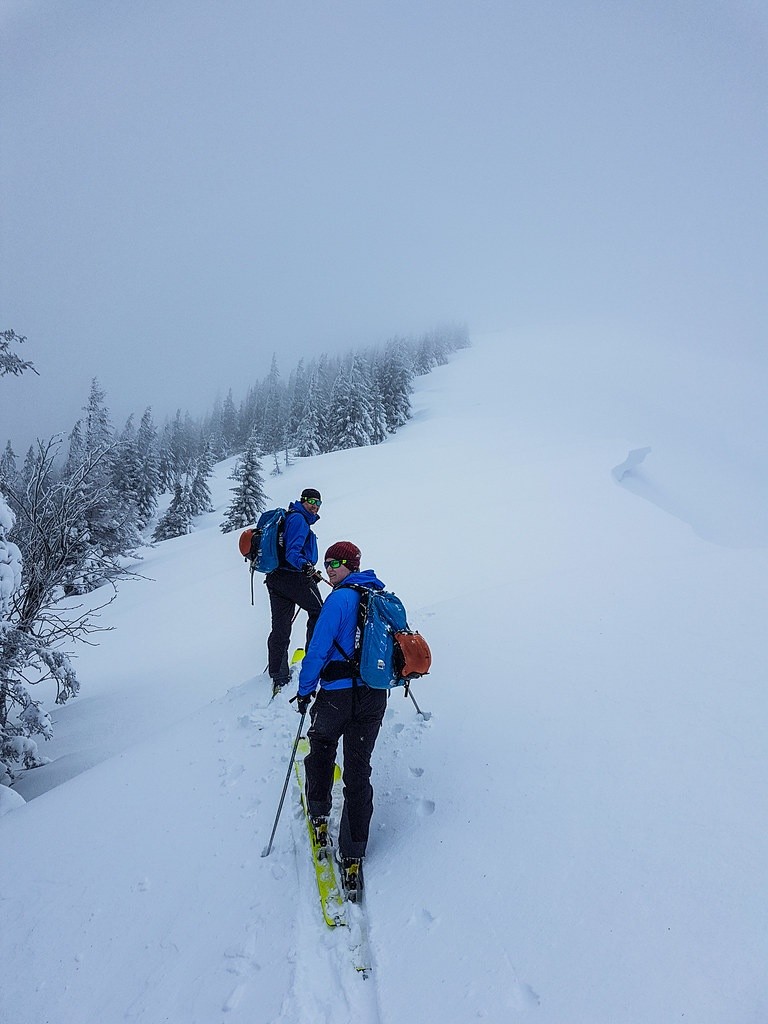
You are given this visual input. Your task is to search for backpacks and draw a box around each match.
[329,584,432,697]
[238,508,311,573]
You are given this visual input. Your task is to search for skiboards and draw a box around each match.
[266,647,374,984]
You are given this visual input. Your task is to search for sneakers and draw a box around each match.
[273,667,296,694]
[308,814,329,845]
[335,847,360,893]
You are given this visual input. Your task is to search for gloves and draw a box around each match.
[313,571,323,583]
[288,691,316,714]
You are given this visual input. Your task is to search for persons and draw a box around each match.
[288,540,389,895]
[263,488,324,694]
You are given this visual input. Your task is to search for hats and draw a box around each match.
[325,541,362,574]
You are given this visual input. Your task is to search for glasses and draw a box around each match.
[305,497,322,506]
[324,560,347,569]
[301,489,321,502]
